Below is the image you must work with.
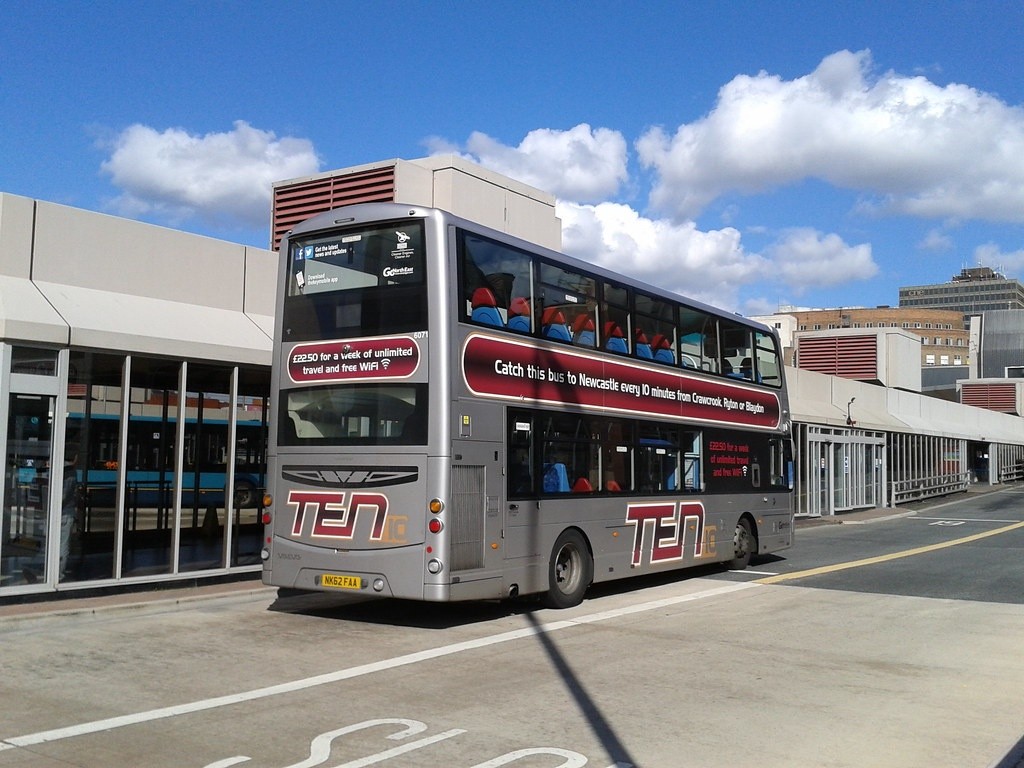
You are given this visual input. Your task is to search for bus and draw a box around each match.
[12,384,266,511]
[262,202,796,608]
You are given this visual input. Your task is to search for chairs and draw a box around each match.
[507,299,530,332]
[541,305,573,343]
[651,332,675,362]
[570,314,594,346]
[636,328,654,360]
[715,358,733,375]
[605,322,626,353]
[543,462,570,491]
[470,288,504,329]
[740,357,762,382]
